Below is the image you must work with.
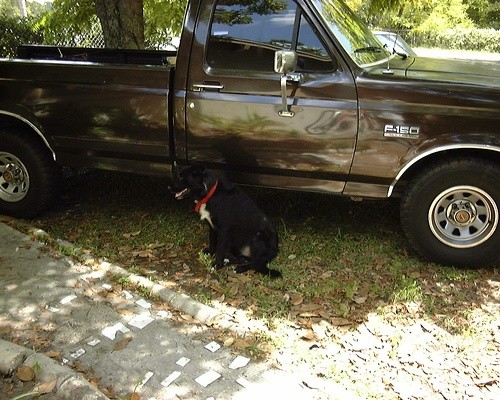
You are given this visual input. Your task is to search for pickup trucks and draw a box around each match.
[1,0,500,271]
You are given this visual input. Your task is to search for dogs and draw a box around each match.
[167,161,285,280]
